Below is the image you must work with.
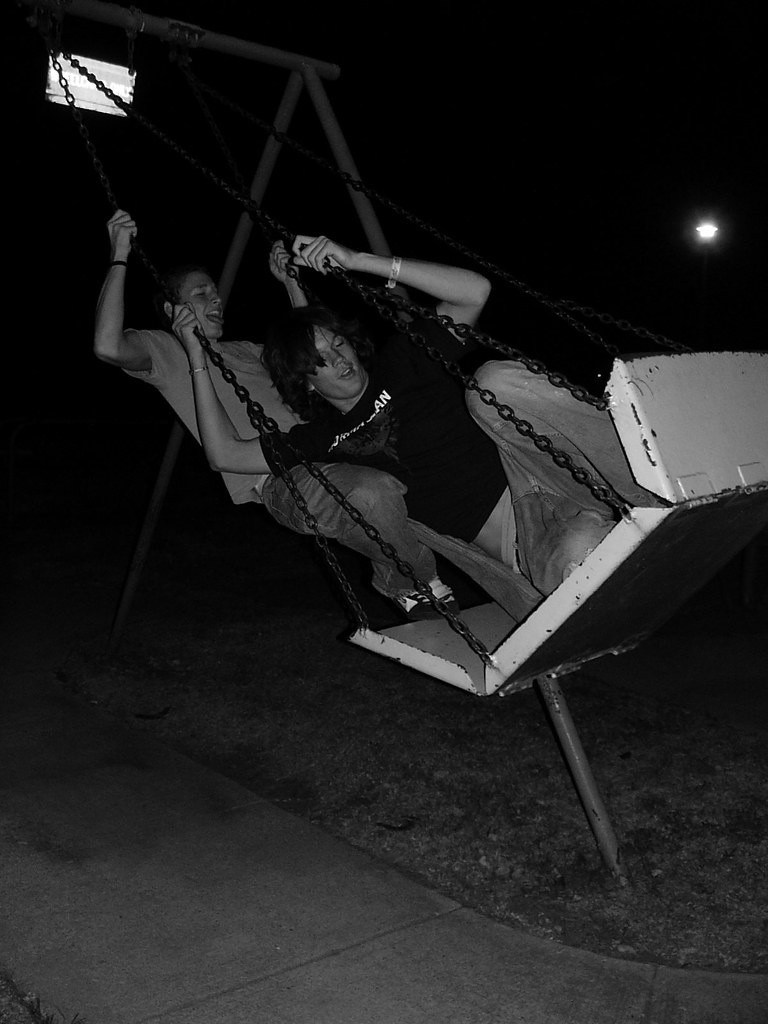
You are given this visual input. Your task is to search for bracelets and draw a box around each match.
[110,261,128,268]
[189,366,209,375]
[388,257,402,289]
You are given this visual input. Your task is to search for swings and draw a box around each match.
[41,33,768,699]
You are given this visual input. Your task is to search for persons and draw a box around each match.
[170,233,672,596]
[92,208,461,620]
